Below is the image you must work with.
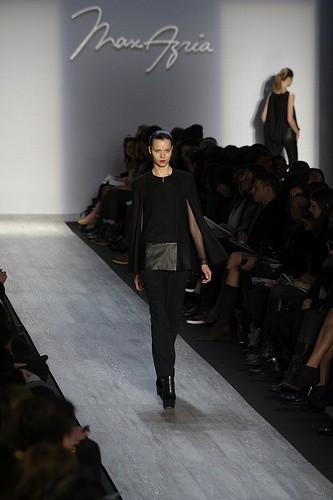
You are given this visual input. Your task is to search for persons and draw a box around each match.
[127,129,229,410]
[77,124,333,435]
[260,68,300,172]
[0,269,108,500]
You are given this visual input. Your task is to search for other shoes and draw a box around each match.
[282,365,319,392]
[157,377,176,409]
[92,238,110,246]
[182,304,200,318]
[247,364,266,375]
[87,232,98,239]
[265,373,282,382]
[112,255,129,264]
[186,312,208,325]
[318,422,333,435]
[80,225,96,232]
[78,218,97,225]
[109,238,128,253]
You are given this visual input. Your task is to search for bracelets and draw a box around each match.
[199,258,209,265]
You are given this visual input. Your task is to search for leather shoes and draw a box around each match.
[294,343,313,363]
[279,390,307,404]
[271,379,287,393]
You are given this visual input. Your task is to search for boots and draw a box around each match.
[196,267,230,323]
[198,284,239,343]
[242,328,263,354]
[255,295,288,366]
